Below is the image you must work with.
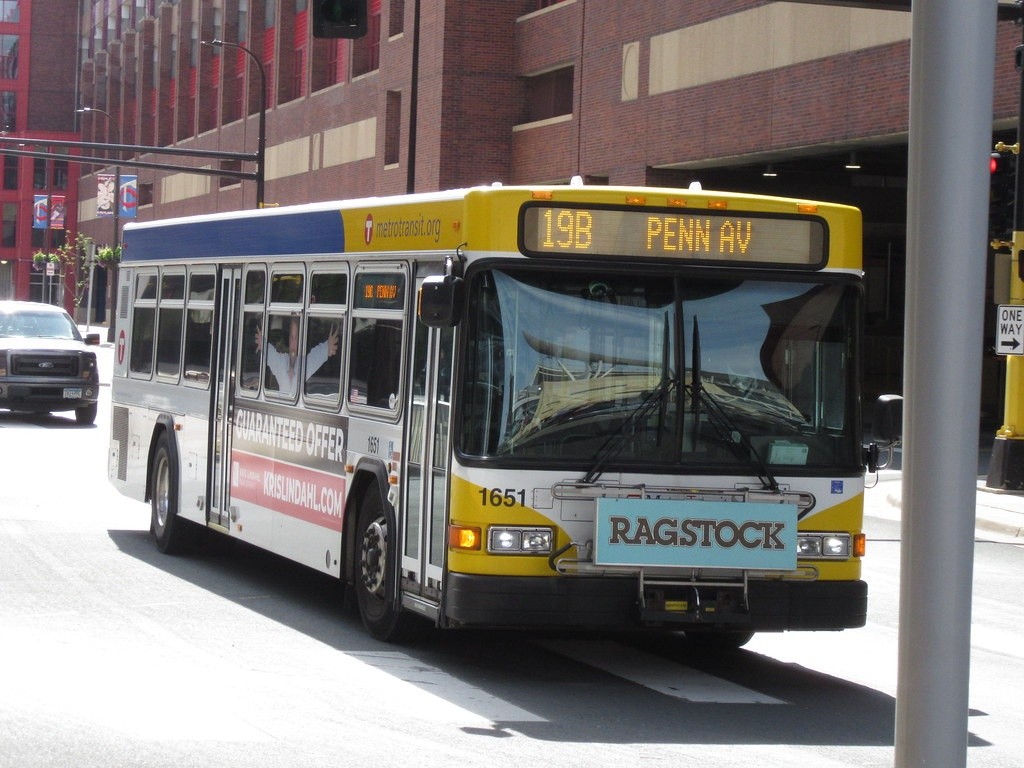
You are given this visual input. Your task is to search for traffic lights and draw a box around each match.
[313,0,367,38]
[990,150,1010,215]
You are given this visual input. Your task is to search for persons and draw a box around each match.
[254,317,339,396]
[703,342,790,419]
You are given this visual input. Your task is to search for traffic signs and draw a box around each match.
[995,304,1024,356]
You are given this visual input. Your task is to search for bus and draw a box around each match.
[109,174,904,642]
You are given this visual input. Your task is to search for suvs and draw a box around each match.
[0,301,100,423]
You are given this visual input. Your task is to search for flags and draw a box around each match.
[33,195,64,229]
[96,174,137,218]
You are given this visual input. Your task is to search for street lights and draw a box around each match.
[200,39,265,208]
[75,106,121,342]
[19,143,50,302]
